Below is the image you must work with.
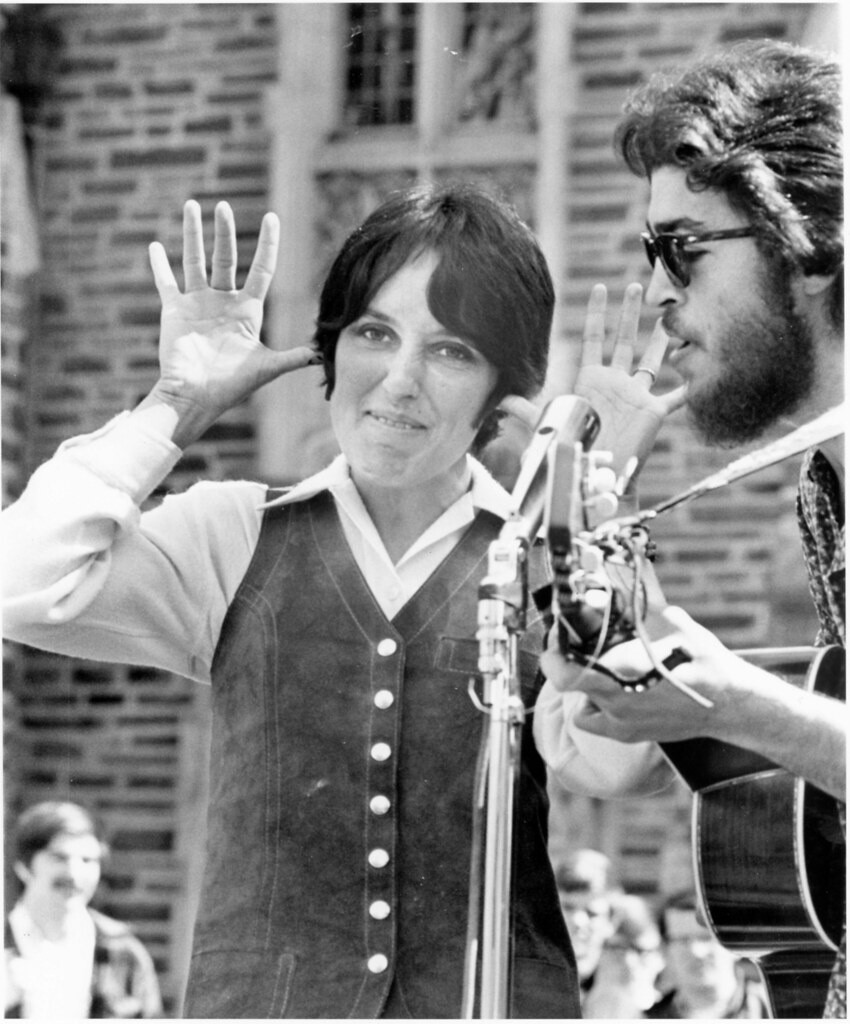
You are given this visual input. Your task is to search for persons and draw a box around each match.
[2,800,167,1019]
[555,847,773,1018]
[534,38,846,1019]
[2,182,687,1019]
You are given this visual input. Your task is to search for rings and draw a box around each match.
[633,370,656,383]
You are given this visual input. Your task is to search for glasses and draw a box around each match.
[642,227,765,286]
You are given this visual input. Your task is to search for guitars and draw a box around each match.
[526,432,846,1018]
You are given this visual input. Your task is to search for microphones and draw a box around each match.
[499,395,602,553]
